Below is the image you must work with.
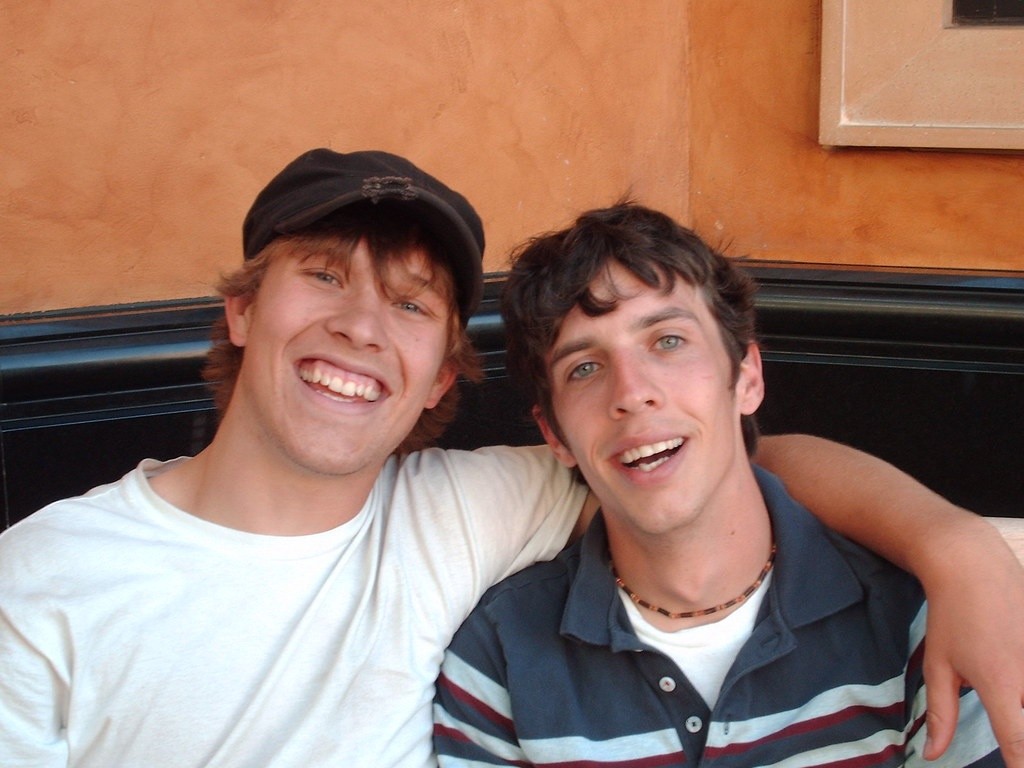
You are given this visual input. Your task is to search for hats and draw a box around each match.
[241,148,487,327]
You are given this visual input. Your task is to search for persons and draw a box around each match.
[2,148,1024,767]
[431,185,1024,767]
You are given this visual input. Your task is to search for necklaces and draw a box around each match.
[606,530,777,619]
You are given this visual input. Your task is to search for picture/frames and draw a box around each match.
[818,0,1024,157]
